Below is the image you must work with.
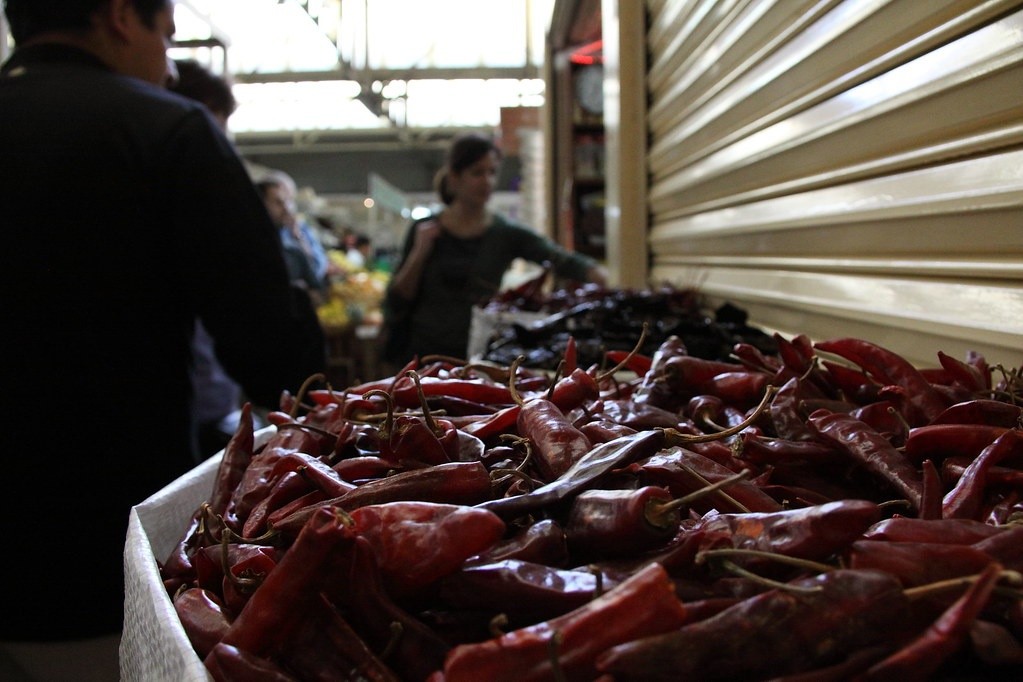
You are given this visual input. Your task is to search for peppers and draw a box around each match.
[163,260,1023,682]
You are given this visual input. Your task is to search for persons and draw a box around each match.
[245,165,398,308]
[0,0,333,682]
[167,55,235,469]
[372,135,608,373]
